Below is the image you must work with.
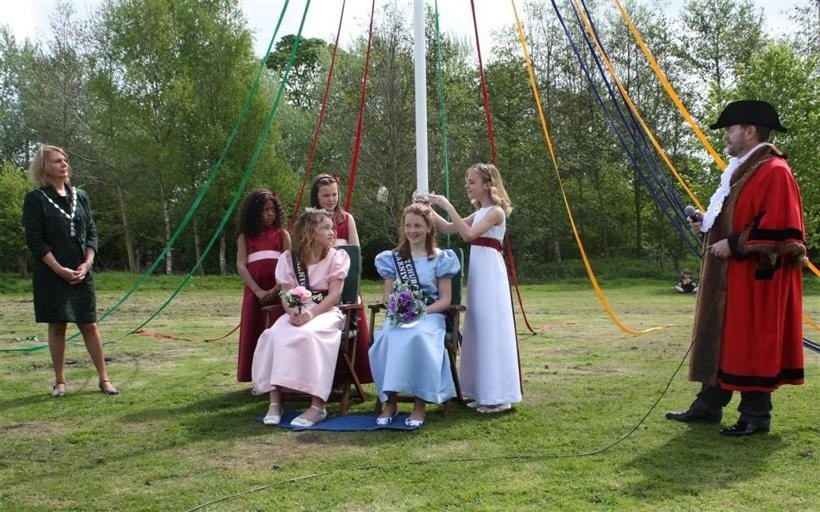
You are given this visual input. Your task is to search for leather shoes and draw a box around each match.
[262,402,285,425]
[718,412,771,436]
[376,404,400,426]
[476,404,511,414]
[290,405,328,428]
[404,411,426,427]
[665,397,723,423]
[466,399,480,408]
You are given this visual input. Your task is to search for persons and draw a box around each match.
[21,143,119,398]
[309,172,375,397]
[674,270,698,294]
[250,208,352,429]
[414,161,524,414]
[664,99,809,437]
[235,188,292,396]
[367,197,463,427]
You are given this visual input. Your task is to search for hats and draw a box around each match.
[709,99,788,134]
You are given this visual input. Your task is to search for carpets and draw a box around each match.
[256,413,418,431]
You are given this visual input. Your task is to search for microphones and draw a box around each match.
[684,205,704,234]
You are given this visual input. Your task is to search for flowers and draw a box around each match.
[382,278,428,329]
[280,286,313,315]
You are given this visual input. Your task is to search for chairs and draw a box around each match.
[368,248,466,418]
[262,246,367,415]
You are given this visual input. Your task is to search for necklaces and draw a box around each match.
[35,185,79,238]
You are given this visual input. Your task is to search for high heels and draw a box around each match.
[99,380,119,395]
[52,381,67,397]
